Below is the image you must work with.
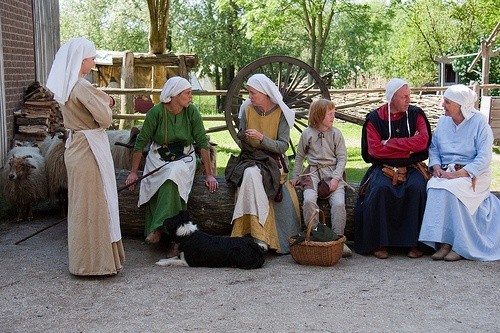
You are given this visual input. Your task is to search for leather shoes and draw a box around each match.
[373,247,388,259]
[407,248,423,258]
[340,244,352,257]
[166,240,181,258]
[144,228,161,245]
[432,243,461,260]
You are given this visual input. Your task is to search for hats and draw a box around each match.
[443,84,477,120]
[159,76,191,103]
[386,78,410,107]
[237,74,295,129]
[45,37,96,105]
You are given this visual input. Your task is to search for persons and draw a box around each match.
[125,75,220,261]
[230,74,301,254]
[45,37,125,275]
[418,84,500,261]
[289,98,353,257]
[352,78,433,259]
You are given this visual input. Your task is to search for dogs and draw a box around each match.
[146,210,267,269]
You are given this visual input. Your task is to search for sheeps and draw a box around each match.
[1,127,218,222]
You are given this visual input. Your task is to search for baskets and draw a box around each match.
[287,209,347,266]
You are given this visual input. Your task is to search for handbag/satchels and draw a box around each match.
[378,161,409,187]
[417,163,433,179]
